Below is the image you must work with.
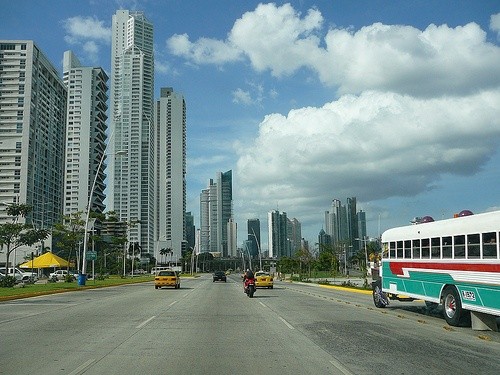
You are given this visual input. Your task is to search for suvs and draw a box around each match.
[0,266,39,284]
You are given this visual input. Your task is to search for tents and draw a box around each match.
[18,251,75,268]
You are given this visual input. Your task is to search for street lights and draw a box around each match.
[81,133,126,273]
[238,227,262,271]
[339,244,353,276]
[355,236,375,276]
[197,228,201,254]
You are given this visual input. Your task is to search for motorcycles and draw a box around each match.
[241,276,256,298]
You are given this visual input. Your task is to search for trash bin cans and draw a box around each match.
[78,274,87,286]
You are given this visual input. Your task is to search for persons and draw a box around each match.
[242,268,257,292]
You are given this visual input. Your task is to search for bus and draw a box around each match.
[369,210,500,328]
[150,266,182,277]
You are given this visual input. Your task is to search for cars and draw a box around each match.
[48,269,79,282]
[153,269,180,289]
[213,271,226,282]
[129,269,146,275]
[254,270,274,289]
